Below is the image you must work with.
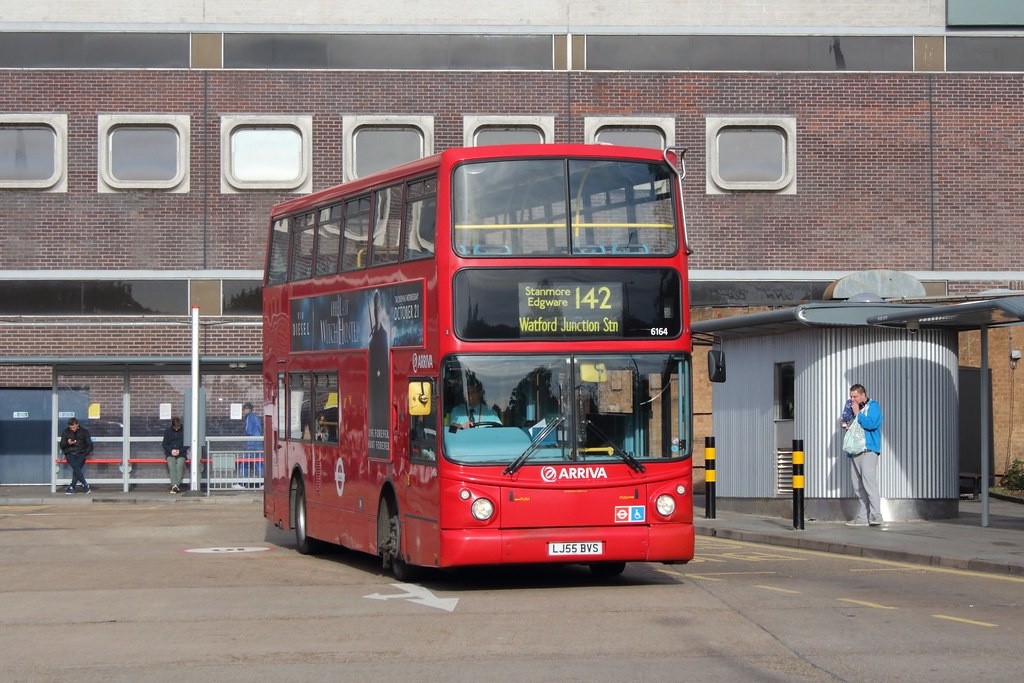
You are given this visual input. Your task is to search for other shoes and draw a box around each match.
[170,485,179,493]
[82,483,90,494]
[233,484,245,489]
[261,485,264,489]
[65,484,74,494]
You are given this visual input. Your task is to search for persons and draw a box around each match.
[840,384,885,526]
[163,417,188,495]
[450,380,503,431]
[61,417,92,494]
[232,402,266,488]
[367,290,386,429]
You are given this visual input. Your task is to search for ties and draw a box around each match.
[469,409,475,428]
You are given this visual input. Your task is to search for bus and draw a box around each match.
[257,141,727,587]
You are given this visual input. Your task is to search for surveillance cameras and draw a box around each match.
[906,320,919,333]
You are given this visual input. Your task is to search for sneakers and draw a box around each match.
[845,516,870,526]
[869,513,883,524]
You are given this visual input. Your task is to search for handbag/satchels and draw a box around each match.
[842,412,867,456]
